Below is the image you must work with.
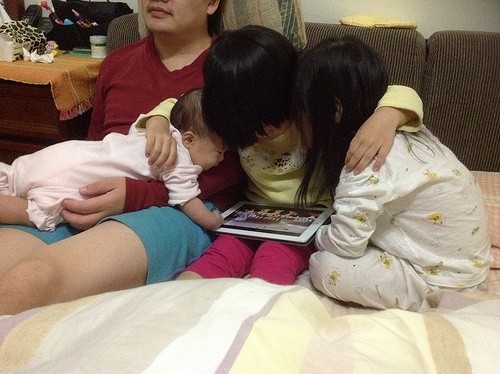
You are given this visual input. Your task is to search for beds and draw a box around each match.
[0,166,500,374]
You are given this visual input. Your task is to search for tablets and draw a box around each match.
[212,200,335,244]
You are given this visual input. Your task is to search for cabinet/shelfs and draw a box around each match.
[0,56,106,165]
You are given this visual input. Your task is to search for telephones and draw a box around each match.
[20,5,56,41]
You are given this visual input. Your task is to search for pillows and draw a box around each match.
[138,0,306,52]
[303,21,426,97]
[105,13,141,54]
[420,30,499,172]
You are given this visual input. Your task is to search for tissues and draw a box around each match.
[0,21,47,62]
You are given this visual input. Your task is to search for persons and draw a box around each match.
[134,25,423,286]
[0,88,232,231]
[0,0,243,316]
[291,35,491,312]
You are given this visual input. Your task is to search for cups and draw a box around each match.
[90,36,107,58]
